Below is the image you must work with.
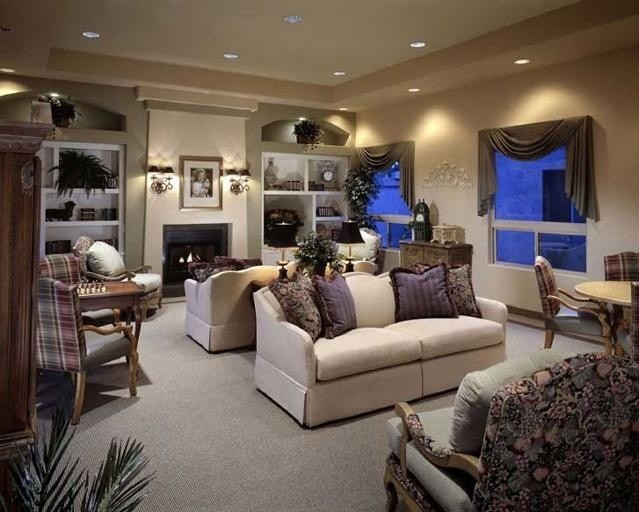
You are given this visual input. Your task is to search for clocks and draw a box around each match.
[412,196,433,240]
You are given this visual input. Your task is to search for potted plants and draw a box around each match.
[293,118,326,153]
[44,95,87,130]
[46,150,117,200]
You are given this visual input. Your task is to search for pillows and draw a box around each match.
[85,236,129,275]
[442,261,481,320]
[267,265,321,340]
[389,262,454,322]
[188,253,244,283]
[313,274,355,335]
[213,253,263,271]
[444,345,581,455]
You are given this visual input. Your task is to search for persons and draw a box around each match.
[190,167,213,198]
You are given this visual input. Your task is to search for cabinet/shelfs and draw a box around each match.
[33,134,127,267]
[398,239,475,276]
[1,114,56,505]
[257,150,353,269]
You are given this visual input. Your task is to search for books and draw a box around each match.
[317,206,336,217]
[282,180,325,192]
[45,240,72,255]
[105,238,118,251]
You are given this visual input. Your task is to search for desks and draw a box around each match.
[71,278,148,384]
[573,279,636,309]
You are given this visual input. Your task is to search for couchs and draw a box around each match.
[177,252,379,356]
[247,261,509,430]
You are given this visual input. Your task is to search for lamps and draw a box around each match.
[336,216,365,272]
[270,218,296,283]
[228,166,252,196]
[146,162,174,194]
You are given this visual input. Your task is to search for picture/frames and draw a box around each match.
[176,154,226,212]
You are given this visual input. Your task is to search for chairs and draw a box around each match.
[342,228,382,272]
[605,250,638,342]
[385,342,638,511]
[71,234,165,322]
[33,275,141,425]
[529,258,623,357]
[613,281,639,359]
[35,249,123,327]
